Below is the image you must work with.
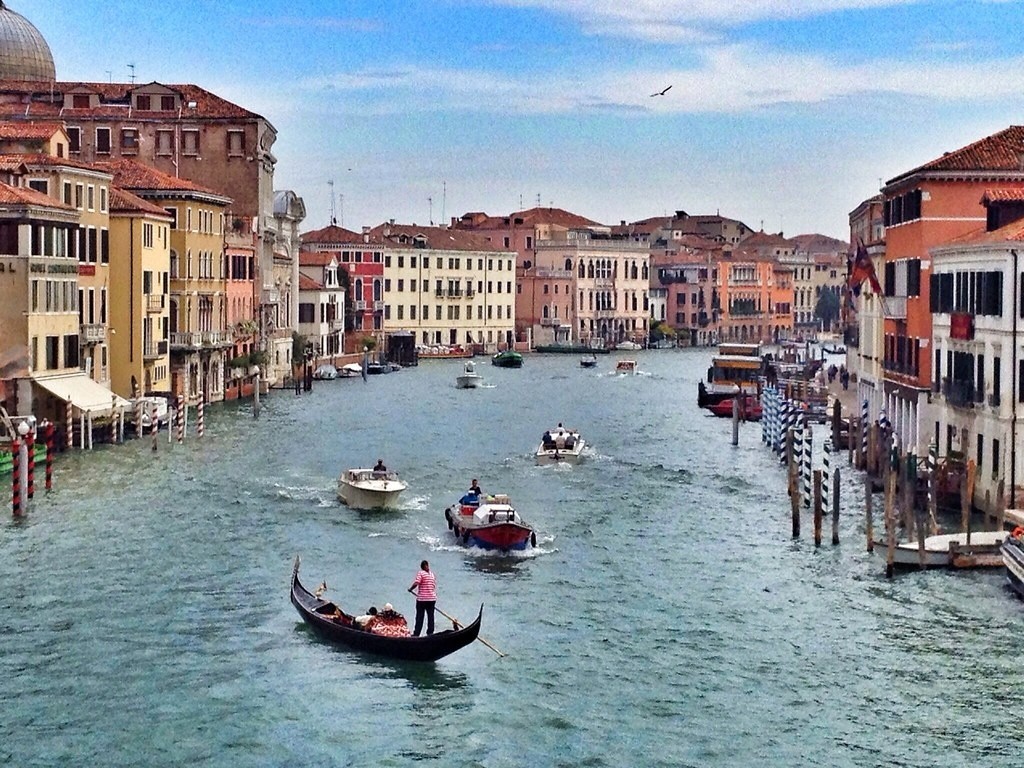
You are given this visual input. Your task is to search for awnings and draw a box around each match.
[35,373,132,413]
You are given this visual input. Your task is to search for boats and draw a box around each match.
[698,339,847,422]
[457,361,483,388]
[536,345,609,354]
[1,444,48,475]
[129,394,179,432]
[872,531,1024,601]
[615,360,637,375]
[337,467,408,511]
[580,356,597,367]
[445,491,537,552]
[616,341,642,350]
[536,430,586,464]
[313,363,362,380]
[492,350,523,368]
[290,554,484,661]
[649,340,676,349]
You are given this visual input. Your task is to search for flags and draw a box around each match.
[848,247,875,289]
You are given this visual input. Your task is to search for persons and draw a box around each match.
[828,364,845,383]
[542,431,556,449]
[469,479,481,497]
[842,369,849,390]
[408,560,438,637]
[554,423,566,433]
[565,432,576,449]
[372,459,386,480]
[556,432,565,449]
[355,607,377,626]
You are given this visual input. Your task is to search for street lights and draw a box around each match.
[252,366,260,417]
[17,421,30,516]
[732,384,740,446]
[363,347,369,380]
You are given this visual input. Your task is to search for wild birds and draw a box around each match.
[649,85,673,97]
